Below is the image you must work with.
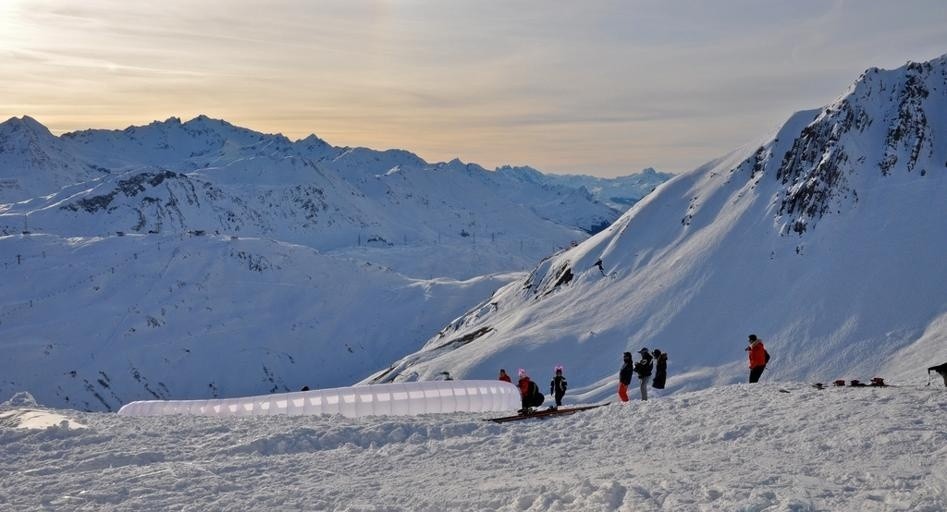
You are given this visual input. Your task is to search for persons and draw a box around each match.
[516,368,530,410]
[652,349,667,389]
[744,334,770,383]
[634,348,654,401]
[550,367,567,406]
[618,352,633,402]
[498,369,511,382]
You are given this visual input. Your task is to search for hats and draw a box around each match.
[637,347,649,354]
